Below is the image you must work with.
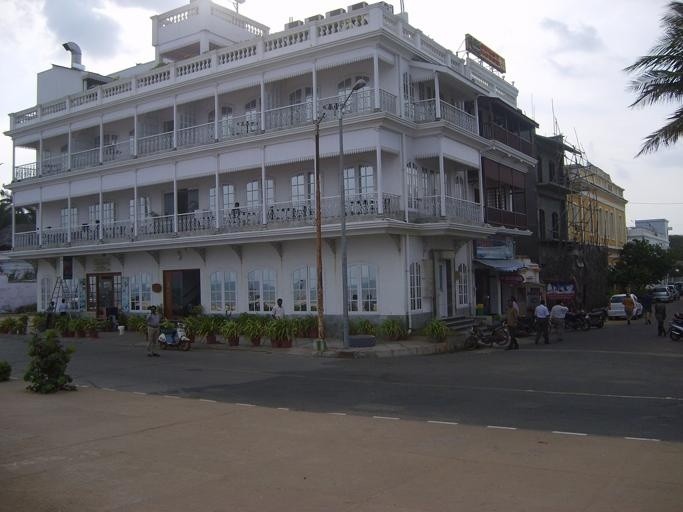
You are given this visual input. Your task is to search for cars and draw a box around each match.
[607,294,643,320]
[649,282,682,307]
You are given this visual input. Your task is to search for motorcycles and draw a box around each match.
[518,309,604,336]
[464,319,513,352]
[158,321,191,352]
[666,312,682,343]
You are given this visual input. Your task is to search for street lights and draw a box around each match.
[312,102,344,350]
[339,79,366,346]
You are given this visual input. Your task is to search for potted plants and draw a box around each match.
[0,310,128,339]
[181,305,454,348]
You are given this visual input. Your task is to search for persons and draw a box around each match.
[44,301,55,313]
[144,305,161,359]
[57,298,68,316]
[228,202,248,225]
[622,290,668,338]
[550,300,569,342]
[271,298,286,321]
[510,295,520,317]
[533,299,551,346]
[94,219,105,231]
[504,300,519,352]
